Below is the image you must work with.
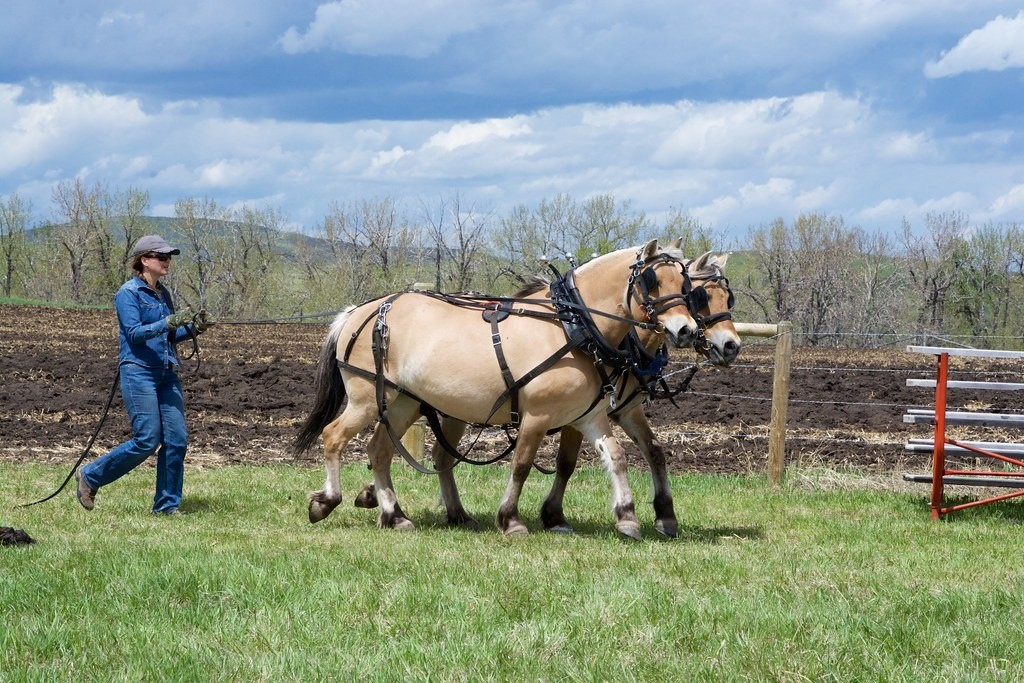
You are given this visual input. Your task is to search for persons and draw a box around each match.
[76,235,218,517]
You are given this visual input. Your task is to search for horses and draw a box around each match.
[292,236,741,541]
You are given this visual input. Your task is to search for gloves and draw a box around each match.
[167,306,194,330]
[192,309,217,333]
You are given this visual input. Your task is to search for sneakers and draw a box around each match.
[171,508,186,516]
[75,465,97,510]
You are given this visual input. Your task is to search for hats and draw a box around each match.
[131,235,180,257]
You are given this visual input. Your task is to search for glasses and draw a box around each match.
[142,253,171,261]
